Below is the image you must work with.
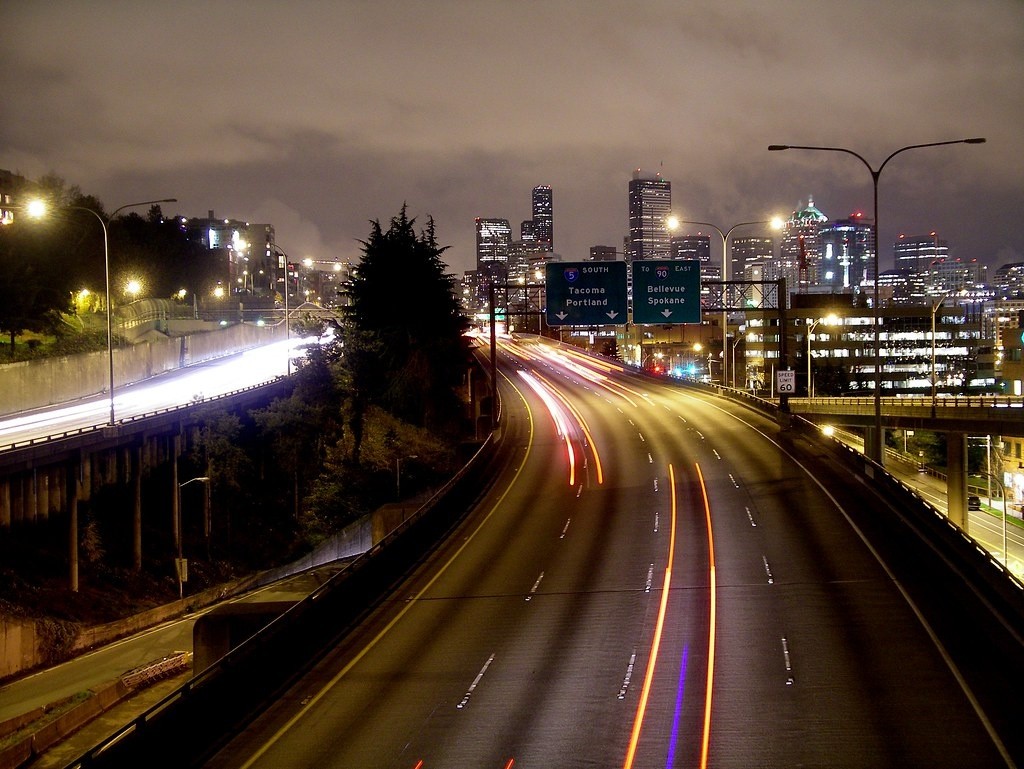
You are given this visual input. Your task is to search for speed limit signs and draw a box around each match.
[777,371,796,394]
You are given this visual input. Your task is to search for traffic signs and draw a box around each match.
[545,260,628,325]
[632,259,702,326]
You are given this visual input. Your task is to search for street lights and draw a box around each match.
[769,137,988,465]
[176,475,211,600]
[667,217,785,385]
[236,240,291,377]
[535,270,543,338]
[932,289,967,404]
[26,196,180,424]
[807,314,838,397]
[971,472,1008,570]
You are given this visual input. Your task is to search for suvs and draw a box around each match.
[968,493,981,511]
